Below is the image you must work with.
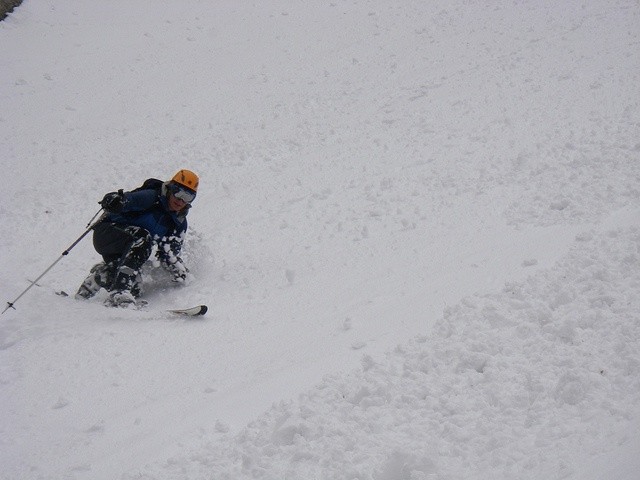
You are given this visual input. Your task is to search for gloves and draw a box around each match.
[102,192,125,212]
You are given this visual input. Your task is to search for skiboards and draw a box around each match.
[26,278,207,317]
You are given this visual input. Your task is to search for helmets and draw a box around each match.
[172,170,199,192]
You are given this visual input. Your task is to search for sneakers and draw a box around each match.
[79,264,101,299]
[108,290,136,306]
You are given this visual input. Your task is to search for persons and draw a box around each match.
[77,170,198,309]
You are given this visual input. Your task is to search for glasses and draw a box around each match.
[165,185,196,205]
